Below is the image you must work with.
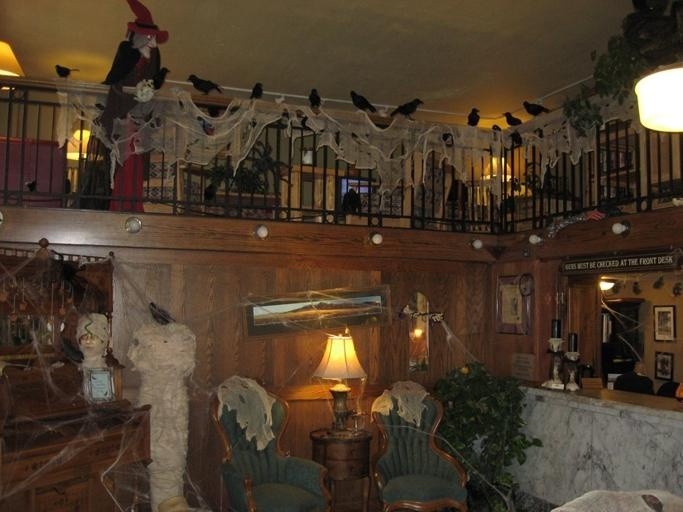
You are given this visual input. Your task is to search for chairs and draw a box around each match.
[369,380,468,512]
[656,382,682,399]
[575,363,608,389]
[614,372,655,395]
[206,374,333,512]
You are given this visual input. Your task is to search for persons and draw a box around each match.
[75,313,110,366]
[78,0,170,211]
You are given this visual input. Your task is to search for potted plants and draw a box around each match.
[432,361,544,512]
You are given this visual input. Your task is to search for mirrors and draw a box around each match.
[567,269,682,409]
[407,291,430,374]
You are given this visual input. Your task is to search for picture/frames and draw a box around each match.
[653,350,674,381]
[493,274,531,335]
[652,304,676,342]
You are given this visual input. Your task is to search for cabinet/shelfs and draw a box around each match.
[600,297,645,373]
[0,236,154,512]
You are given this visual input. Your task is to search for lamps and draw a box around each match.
[467,236,482,251]
[367,231,382,249]
[611,220,629,237]
[632,60,682,133]
[527,234,543,246]
[598,275,616,291]
[124,216,144,235]
[253,223,268,241]
[311,335,368,436]
[0,40,26,90]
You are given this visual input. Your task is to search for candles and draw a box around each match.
[567,332,578,353]
[551,319,561,338]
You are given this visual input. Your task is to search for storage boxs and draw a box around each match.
[0,134,68,208]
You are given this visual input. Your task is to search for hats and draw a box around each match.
[125,0,170,43]
